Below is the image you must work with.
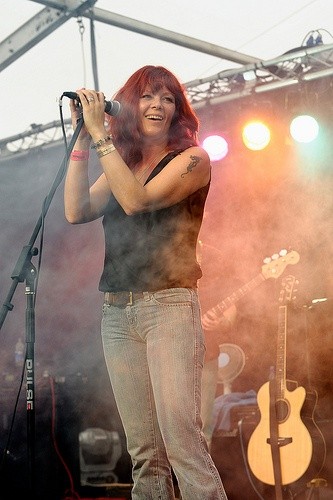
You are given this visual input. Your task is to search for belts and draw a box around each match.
[104,291,148,308]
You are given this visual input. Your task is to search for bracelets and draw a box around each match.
[91,134,113,149]
[96,144,116,159]
[71,150,89,162]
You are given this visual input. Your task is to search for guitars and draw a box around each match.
[202,248,301,333]
[245,271,329,492]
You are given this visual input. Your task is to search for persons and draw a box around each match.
[63,64,229,500]
[195,241,237,454]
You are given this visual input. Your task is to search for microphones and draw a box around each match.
[62,92,123,117]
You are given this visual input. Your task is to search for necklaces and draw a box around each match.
[136,147,170,183]
